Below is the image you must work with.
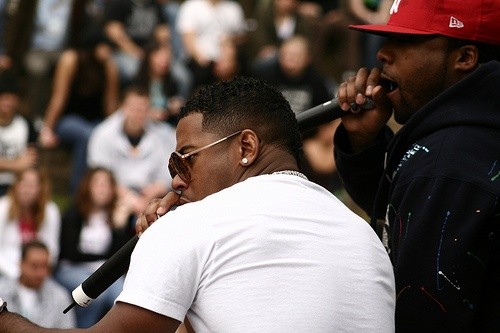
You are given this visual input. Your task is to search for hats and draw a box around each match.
[348,0,500,45]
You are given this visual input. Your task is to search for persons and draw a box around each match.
[0,168,62,275]
[0,77,396,333]
[60,169,137,329]
[333,0,500,333]
[2,0,391,221]
[0,240,76,331]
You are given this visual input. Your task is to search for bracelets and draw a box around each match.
[0,297,9,315]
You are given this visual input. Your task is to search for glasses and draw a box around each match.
[168,130,242,182]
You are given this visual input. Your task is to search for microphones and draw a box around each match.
[64,205,179,314]
[294,95,376,132]
